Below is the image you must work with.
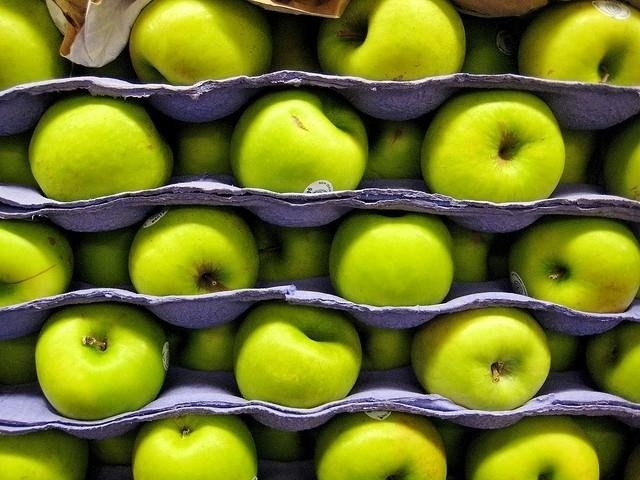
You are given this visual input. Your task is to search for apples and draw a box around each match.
[1,0,640,480]
